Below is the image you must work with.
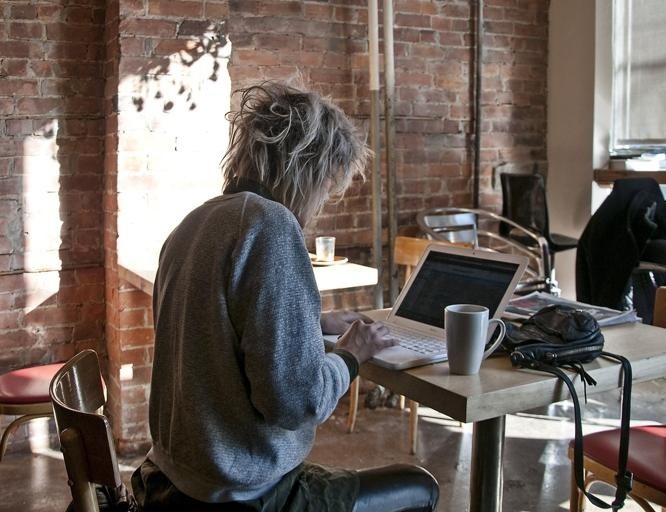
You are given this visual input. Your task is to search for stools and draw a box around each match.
[565,421,665,510]
[1,362,110,464]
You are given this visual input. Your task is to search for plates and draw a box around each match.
[310,255,350,266]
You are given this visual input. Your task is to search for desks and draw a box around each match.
[121,243,382,306]
[316,300,666,511]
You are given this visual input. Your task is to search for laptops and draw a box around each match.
[323,243,530,373]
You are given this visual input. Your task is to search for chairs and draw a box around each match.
[494,172,581,268]
[413,204,555,302]
[337,235,475,454]
[48,350,125,512]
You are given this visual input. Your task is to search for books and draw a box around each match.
[500,291,638,327]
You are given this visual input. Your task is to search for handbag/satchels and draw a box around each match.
[507,303,606,369]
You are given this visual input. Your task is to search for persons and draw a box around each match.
[130,82,440,512]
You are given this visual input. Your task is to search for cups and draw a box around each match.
[314,235,335,261]
[444,304,508,377]
